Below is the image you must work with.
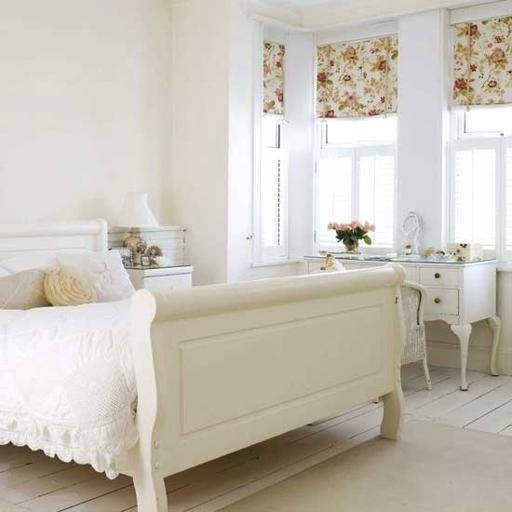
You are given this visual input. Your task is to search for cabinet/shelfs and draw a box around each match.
[302,250,501,393]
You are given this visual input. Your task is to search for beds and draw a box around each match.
[0,219,408,512]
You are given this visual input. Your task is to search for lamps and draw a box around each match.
[110,192,162,263]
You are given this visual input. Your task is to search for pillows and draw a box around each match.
[57,247,136,302]
[0,267,53,312]
[323,252,346,275]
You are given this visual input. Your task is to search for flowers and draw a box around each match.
[326,221,377,250]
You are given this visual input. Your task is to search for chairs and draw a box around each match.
[370,279,432,405]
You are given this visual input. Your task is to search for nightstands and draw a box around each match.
[125,262,193,293]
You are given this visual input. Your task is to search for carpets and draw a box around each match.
[213,416,511,512]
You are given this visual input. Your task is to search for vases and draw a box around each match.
[344,239,361,254]
